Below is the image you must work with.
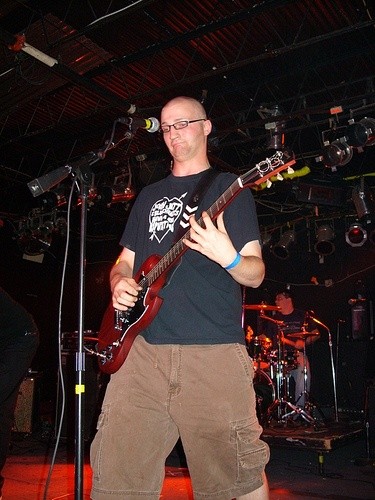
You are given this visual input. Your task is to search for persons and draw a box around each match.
[273,292,320,427]
[90,96,271,500]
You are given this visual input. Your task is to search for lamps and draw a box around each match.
[15,103,375,263]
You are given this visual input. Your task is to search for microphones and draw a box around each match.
[119,116,161,133]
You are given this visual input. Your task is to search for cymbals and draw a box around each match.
[241,304,283,311]
[288,331,320,338]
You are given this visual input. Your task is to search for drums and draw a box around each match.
[270,344,299,372]
[252,369,276,424]
[246,335,272,369]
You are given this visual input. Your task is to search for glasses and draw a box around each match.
[159,119,207,134]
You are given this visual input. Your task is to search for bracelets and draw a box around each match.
[225,253,241,270]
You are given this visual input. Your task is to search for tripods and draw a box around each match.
[260,310,325,428]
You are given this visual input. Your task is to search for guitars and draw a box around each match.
[94,147,297,376]
